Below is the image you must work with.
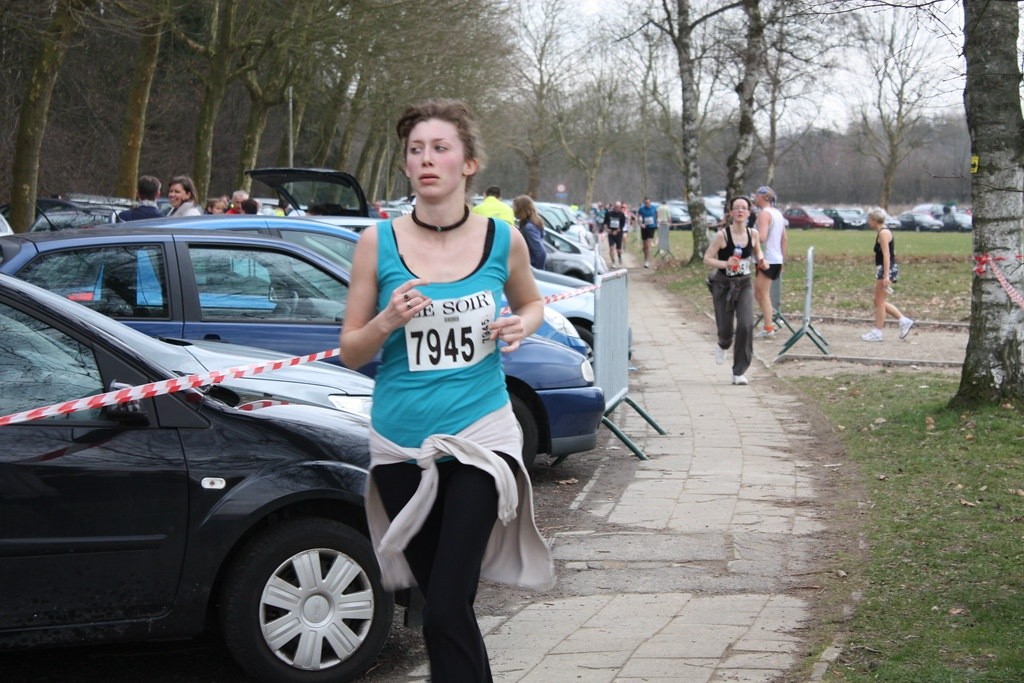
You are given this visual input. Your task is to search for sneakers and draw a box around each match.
[860,332,882,342]
[716,344,728,365]
[899,317,914,339]
[731,374,748,385]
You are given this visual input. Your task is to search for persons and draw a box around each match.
[339,98,544,683]
[115,174,313,224]
[859,209,915,342]
[753,185,787,341]
[621,200,636,252]
[607,203,614,212]
[703,197,770,385]
[637,196,658,268]
[656,201,671,228]
[595,205,609,233]
[513,194,546,270]
[602,201,626,269]
[471,185,515,226]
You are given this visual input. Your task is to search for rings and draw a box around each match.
[404,293,411,301]
[406,301,412,309]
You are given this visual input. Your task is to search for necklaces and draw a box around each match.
[411,202,470,233]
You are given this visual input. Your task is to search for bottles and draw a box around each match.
[730,243,742,271]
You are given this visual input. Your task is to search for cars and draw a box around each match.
[896,213,944,232]
[0,228,606,470]
[660,199,789,230]
[822,208,866,230]
[783,206,834,230]
[0,279,423,683]
[914,204,974,232]
[66,214,596,365]
[27,168,633,361]
[885,214,901,231]
[0,273,377,440]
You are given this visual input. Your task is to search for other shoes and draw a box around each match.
[644,262,648,268]
[619,257,623,265]
[753,330,777,339]
[611,263,616,268]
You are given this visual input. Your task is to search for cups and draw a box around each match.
[883,285,894,294]
